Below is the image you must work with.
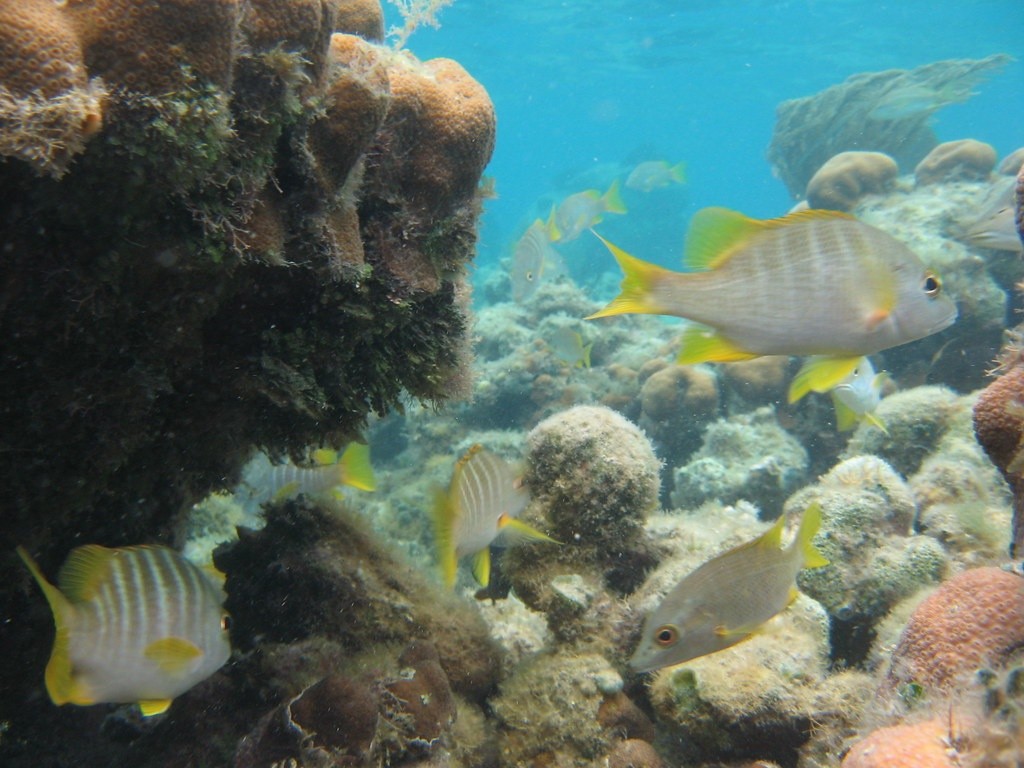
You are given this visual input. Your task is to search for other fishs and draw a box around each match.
[13,542,234,718]
[507,157,1023,435]
[212,440,379,530]
[395,442,564,594]
[630,499,831,675]
